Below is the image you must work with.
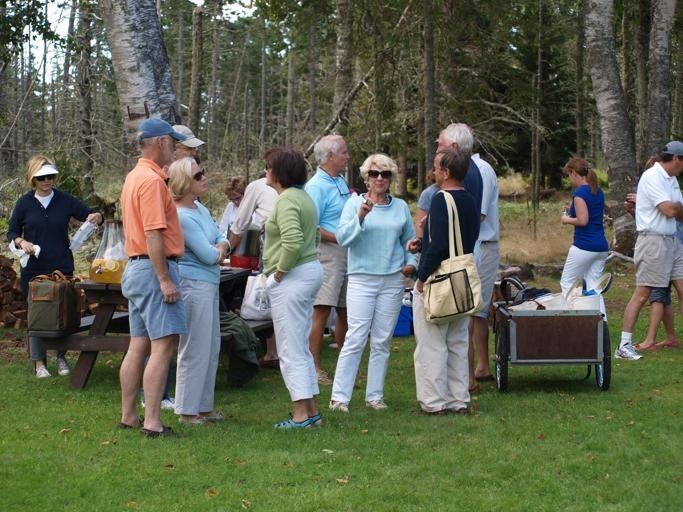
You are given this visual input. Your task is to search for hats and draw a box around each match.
[136,118,188,142]
[172,124,206,148]
[661,141,683,157]
[32,163,60,177]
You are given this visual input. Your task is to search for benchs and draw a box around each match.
[28,311,129,389]
[221,320,273,342]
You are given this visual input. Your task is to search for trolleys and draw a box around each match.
[491,270,616,390]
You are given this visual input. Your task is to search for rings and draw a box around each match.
[632,196,634,201]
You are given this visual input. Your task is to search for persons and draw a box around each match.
[6,155,104,379]
[612,139,682,360]
[218,146,324,428]
[115,119,229,437]
[621,155,680,351]
[304,134,419,412]
[406,123,498,416]
[558,156,610,325]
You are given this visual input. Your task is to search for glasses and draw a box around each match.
[367,170,393,179]
[35,175,55,181]
[193,168,204,181]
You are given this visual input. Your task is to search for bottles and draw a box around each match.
[560,203,569,242]
[89,220,130,286]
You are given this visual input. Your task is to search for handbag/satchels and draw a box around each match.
[239,273,273,322]
[565,273,612,310]
[27,270,82,334]
[422,253,485,325]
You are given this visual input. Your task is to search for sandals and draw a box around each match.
[272,412,323,431]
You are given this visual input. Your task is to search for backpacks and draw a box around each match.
[500,274,550,306]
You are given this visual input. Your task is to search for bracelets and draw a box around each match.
[18,240,24,248]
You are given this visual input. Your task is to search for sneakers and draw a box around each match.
[116,419,143,432]
[179,412,225,426]
[56,356,70,376]
[316,369,333,386]
[634,342,656,350]
[34,365,51,378]
[141,395,175,410]
[256,355,280,369]
[409,409,449,417]
[614,342,644,361]
[365,399,389,409]
[140,426,175,441]
[328,399,349,414]
[469,367,494,395]
[450,407,474,415]
[654,340,680,348]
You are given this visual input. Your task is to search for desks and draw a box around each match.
[69,267,253,390]
